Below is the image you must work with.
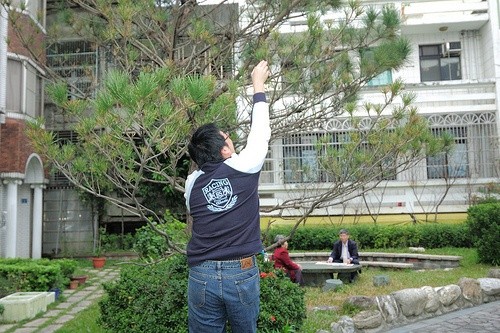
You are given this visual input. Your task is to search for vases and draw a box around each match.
[69,276,87,289]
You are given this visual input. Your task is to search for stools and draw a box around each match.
[325,278,343,292]
[372,276,390,286]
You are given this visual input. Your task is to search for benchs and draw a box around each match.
[266,251,462,271]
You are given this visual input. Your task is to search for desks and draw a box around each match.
[296,263,362,288]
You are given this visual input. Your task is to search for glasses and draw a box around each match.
[224,132,230,141]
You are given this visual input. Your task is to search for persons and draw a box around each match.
[326,229,360,282]
[270,235,303,287]
[183,58,272,333]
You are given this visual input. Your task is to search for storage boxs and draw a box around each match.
[0,289,59,324]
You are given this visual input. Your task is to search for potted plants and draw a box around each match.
[92,233,106,268]
